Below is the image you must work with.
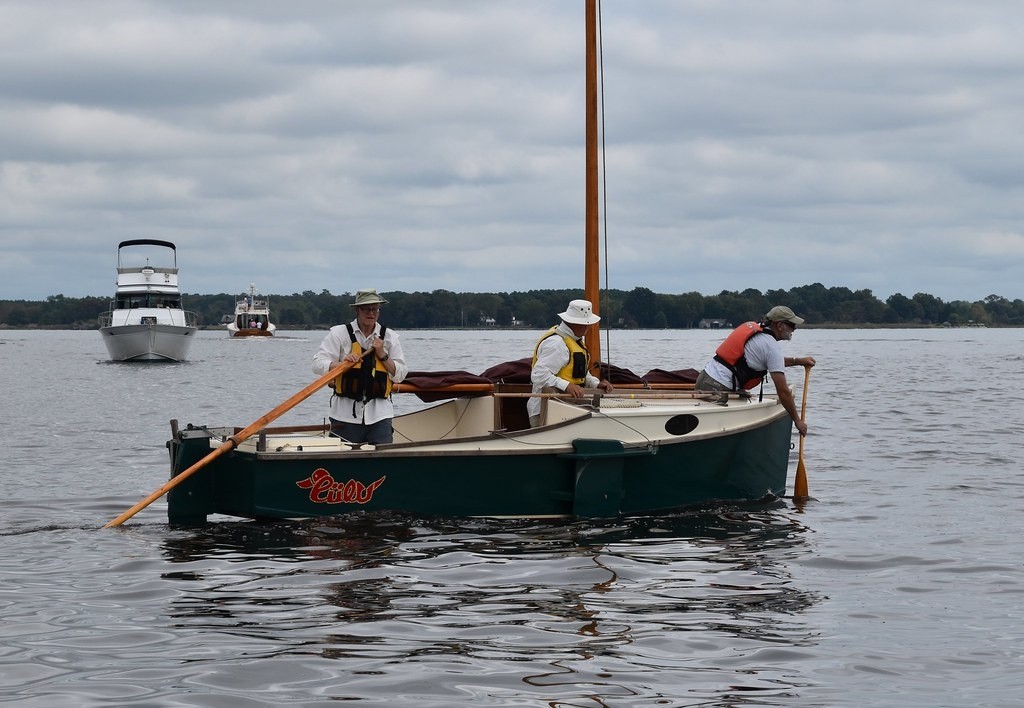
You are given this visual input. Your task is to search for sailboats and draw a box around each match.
[165,0,797,532]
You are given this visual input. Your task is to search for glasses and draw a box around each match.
[357,305,380,313]
[781,320,796,332]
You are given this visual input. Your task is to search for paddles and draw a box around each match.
[792,363,815,498]
[102,346,376,529]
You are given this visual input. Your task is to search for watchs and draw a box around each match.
[380,353,389,361]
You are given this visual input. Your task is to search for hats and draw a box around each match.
[350,288,387,307]
[767,306,805,324]
[557,299,601,325]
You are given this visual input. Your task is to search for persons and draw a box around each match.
[694,304,816,437]
[251,319,262,329]
[526,299,614,429]
[310,288,409,445]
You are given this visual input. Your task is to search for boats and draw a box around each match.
[225,283,277,338]
[97,238,199,364]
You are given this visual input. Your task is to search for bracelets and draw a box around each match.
[793,358,797,366]
